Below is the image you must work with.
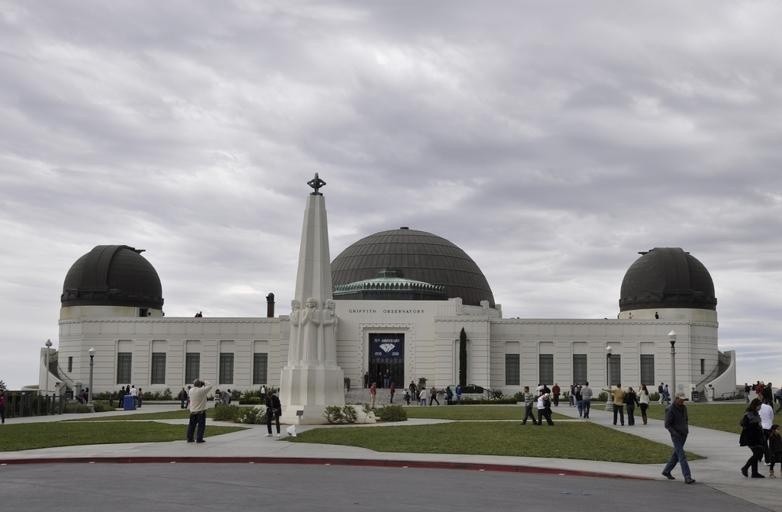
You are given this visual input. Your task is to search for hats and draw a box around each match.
[674,392,688,402]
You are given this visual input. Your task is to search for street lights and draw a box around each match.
[668,328,677,405]
[86,346,96,413]
[606,345,613,411]
[45,339,52,397]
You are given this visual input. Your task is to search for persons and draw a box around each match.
[524,380,782,483]
[0,393,5,423]
[110,383,143,408]
[198,311,202,317]
[76,388,89,405]
[177,378,282,442]
[195,313,198,317]
[343,368,462,409]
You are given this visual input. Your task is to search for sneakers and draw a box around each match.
[662,471,675,480]
[265,433,280,438]
[187,439,205,442]
[742,462,776,479]
[685,478,694,483]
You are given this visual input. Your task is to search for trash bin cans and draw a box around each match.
[123,395,136,410]
[692,391,698,402]
